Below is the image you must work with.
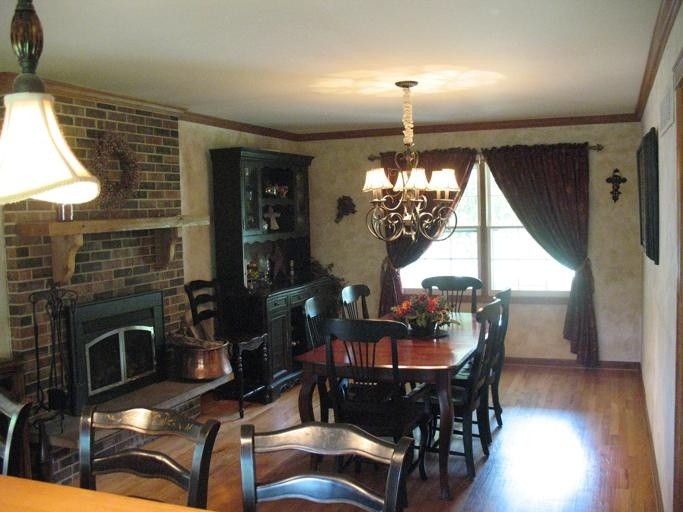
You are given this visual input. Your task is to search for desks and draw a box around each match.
[0,473,215,511]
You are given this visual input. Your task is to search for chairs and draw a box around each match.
[181,277,274,413]
[75,404,220,511]
[240,417,413,511]
[291,273,512,502]
[0,390,32,477]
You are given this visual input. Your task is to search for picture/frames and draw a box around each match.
[637,128,663,265]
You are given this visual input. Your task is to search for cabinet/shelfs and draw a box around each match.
[206,145,313,286]
[212,275,336,407]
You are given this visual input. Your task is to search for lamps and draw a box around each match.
[1,1,102,215]
[355,78,464,241]
